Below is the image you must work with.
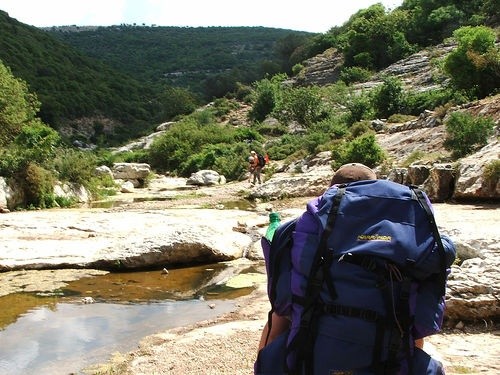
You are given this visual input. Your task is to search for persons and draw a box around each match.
[253,162,456,375]
[248,151,268,185]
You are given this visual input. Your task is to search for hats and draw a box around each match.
[331,163,377,185]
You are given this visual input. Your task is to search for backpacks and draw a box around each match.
[258,154,265,168]
[253,179,447,375]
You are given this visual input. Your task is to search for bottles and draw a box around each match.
[266,212,282,243]
